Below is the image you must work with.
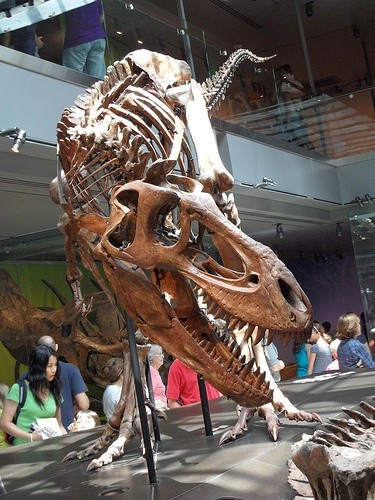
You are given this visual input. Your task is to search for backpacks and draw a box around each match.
[3,379,27,445]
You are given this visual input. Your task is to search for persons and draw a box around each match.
[66,409,100,432]
[21,335,90,433]
[0,0,43,58]
[64,0,107,80]
[292,320,342,378]
[0,344,64,446]
[0,384,12,448]
[166,358,223,409]
[228,89,257,115]
[262,329,285,382]
[335,312,375,369]
[271,69,315,151]
[143,344,168,411]
[101,358,124,421]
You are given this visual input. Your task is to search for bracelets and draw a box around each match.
[31,433,33,442]
